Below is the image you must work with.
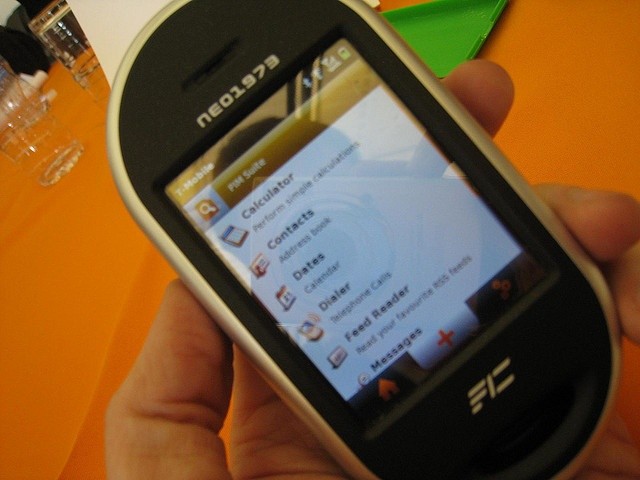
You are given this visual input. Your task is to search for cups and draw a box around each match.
[1,60,84,188]
[28,0,109,113]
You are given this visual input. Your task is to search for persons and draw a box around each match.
[105,59,639,480]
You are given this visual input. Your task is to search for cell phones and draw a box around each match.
[106,0,622,480]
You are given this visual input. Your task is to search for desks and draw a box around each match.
[1,0,640,480]
